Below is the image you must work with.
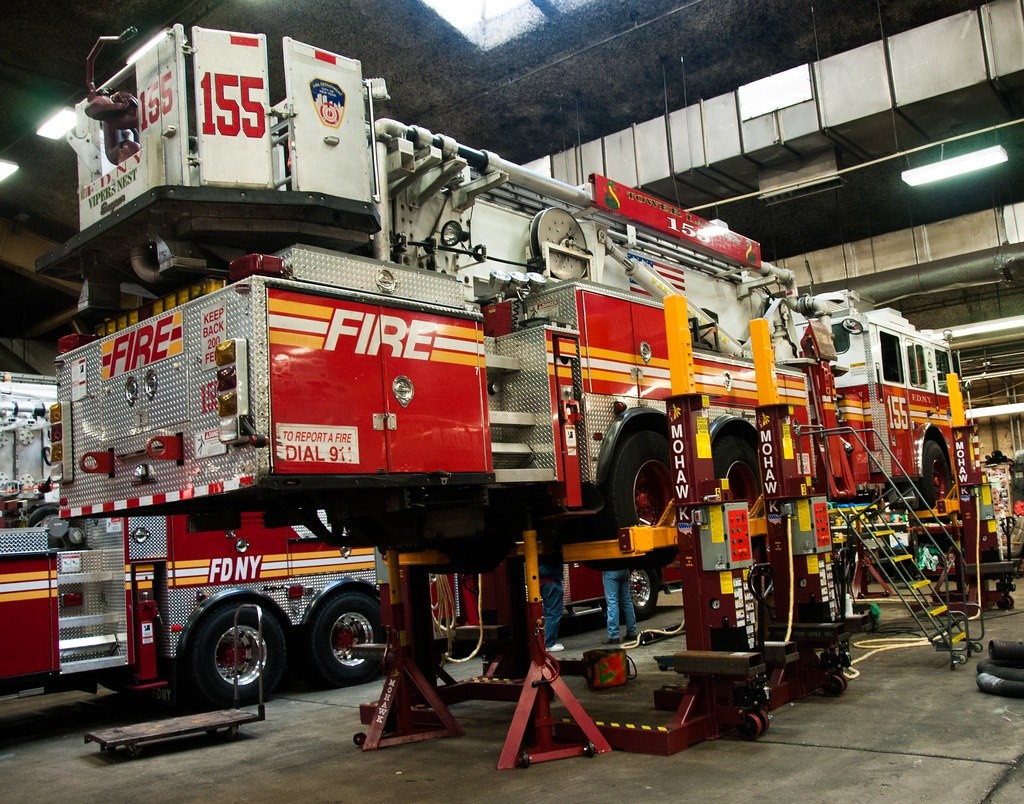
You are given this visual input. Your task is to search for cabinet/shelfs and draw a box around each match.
[909,525,963,582]
[983,465,1013,546]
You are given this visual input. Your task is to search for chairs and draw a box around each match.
[1002,516,1024,558]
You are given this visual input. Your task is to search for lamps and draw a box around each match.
[901,126,1008,187]
[0,158,19,183]
[36,106,75,140]
[934,314,1024,340]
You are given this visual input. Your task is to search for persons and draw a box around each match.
[534,540,568,651]
[601,570,637,644]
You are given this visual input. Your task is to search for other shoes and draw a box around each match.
[602,638,620,644]
[623,635,637,640]
[546,644,565,652]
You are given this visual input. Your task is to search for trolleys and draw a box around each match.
[931,517,1015,611]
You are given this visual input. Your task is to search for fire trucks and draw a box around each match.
[0,23,970,721]
